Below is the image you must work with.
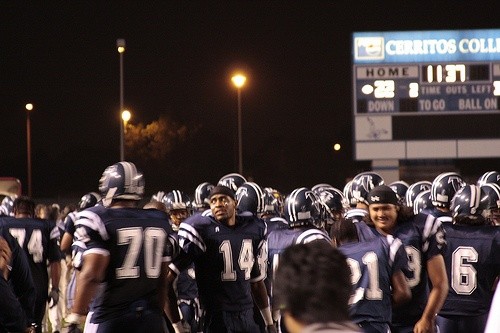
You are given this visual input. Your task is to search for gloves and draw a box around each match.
[60,312,86,333]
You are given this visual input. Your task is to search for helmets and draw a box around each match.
[284,187,313,228]
[163,189,193,213]
[450,184,489,224]
[217,174,247,194]
[477,171,500,187]
[312,183,333,194]
[233,181,264,213]
[413,189,431,215]
[258,187,282,217]
[314,188,349,228]
[192,182,215,207]
[78,192,101,210]
[343,180,351,203]
[476,184,500,216]
[431,172,466,209]
[99,159,145,201]
[350,172,385,206]
[406,181,432,208]
[387,180,409,207]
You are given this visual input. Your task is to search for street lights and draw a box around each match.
[231,72,247,175]
[116,39,130,162]
[25,103,33,197]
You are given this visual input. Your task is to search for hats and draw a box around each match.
[209,184,235,198]
[368,184,398,205]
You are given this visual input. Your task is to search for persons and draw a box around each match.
[274,242,364,333]
[63,162,175,333]
[144,171,500,333]
[0,192,98,333]
